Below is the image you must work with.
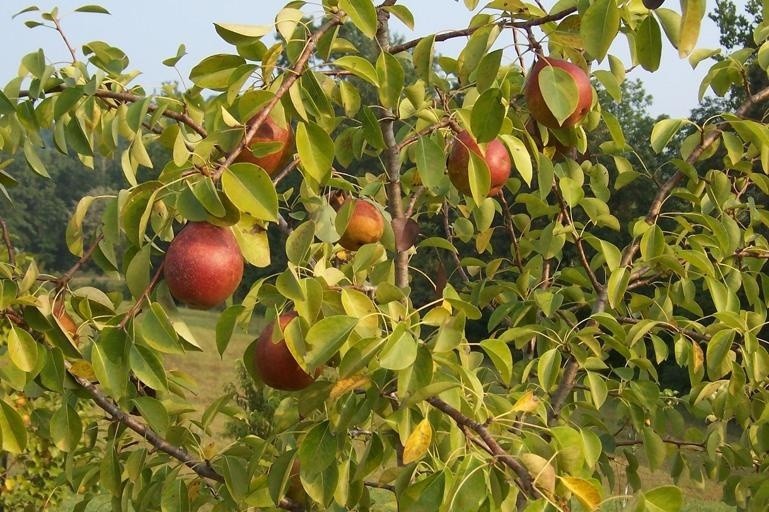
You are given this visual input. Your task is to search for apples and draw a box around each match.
[337,197,384,251]
[163,222,246,309]
[256,309,323,390]
[448,129,512,198]
[528,56,593,129]
[226,109,294,180]
[48,297,79,351]
